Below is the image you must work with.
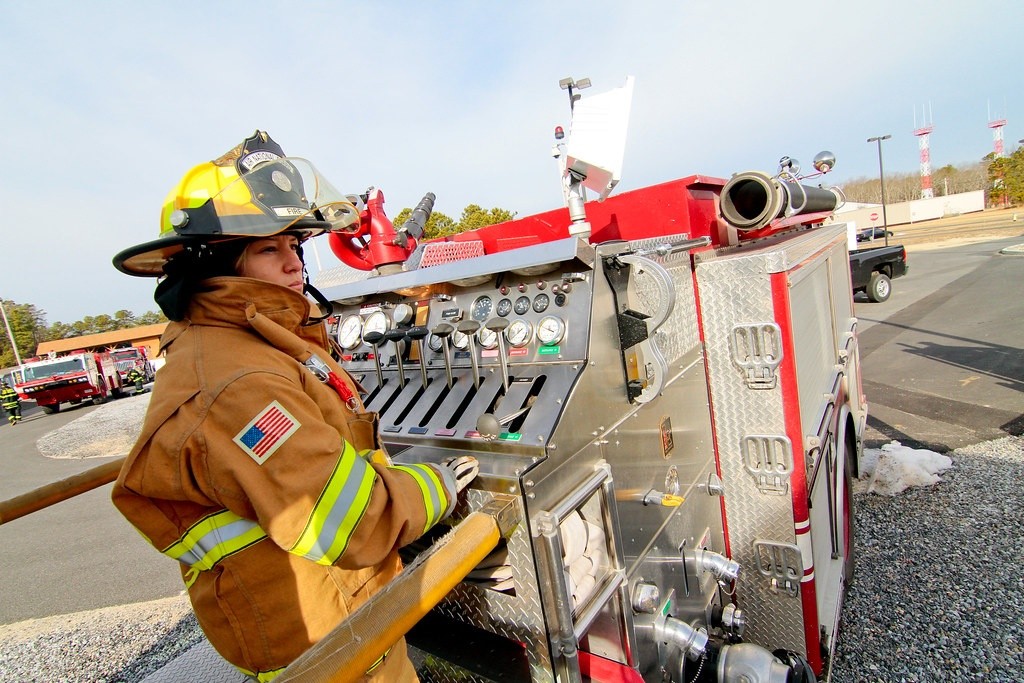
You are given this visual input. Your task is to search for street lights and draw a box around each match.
[865,134,892,246]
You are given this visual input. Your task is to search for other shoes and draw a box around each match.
[11,419,16,426]
[16,418,22,421]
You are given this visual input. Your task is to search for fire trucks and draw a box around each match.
[11,345,156,414]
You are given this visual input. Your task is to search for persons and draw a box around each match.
[127,358,145,393]
[108,128,482,683]
[0,381,22,425]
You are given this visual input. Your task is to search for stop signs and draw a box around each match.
[869,213,879,221]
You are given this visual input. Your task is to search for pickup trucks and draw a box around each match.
[849,244,909,303]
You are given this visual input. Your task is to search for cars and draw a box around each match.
[856,228,894,242]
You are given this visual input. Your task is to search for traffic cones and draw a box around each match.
[1012,213,1017,221]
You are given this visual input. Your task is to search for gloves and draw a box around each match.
[440,456,479,493]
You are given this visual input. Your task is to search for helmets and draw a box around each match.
[111,130,333,277]
[1,381,7,385]
